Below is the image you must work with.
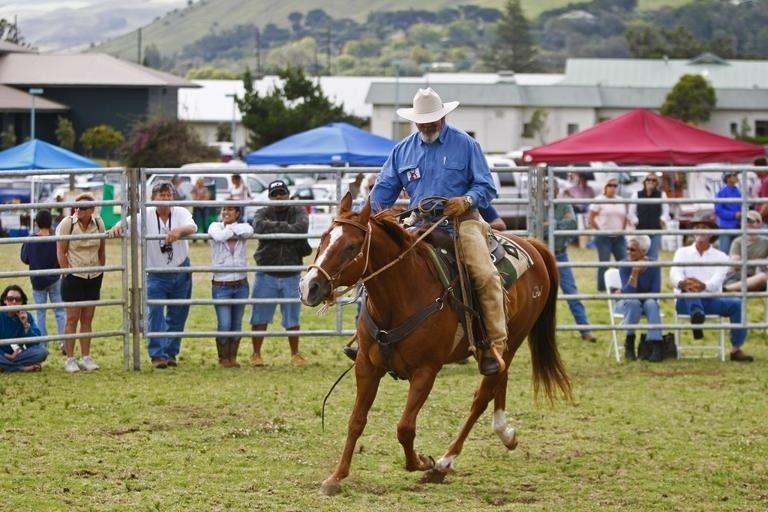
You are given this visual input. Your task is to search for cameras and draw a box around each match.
[160,243,172,253]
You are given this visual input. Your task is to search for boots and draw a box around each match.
[215,340,241,369]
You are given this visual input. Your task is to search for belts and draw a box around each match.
[553,246,567,257]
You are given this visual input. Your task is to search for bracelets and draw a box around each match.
[629,275,637,283]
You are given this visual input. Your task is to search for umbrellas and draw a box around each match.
[246,122,400,216]
[0,136,104,237]
[517,108,765,170]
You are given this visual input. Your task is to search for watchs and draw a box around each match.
[465,195,475,206]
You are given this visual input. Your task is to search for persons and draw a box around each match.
[669,214,756,363]
[109,178,199,370]
[250,178,314,370]
[613,235,666,363]
[1,284,50,375]
[54,194,108,374]
[531,179,601,345]
[206,204,255,368]
[19,208,68,351]
[341,86,503,378]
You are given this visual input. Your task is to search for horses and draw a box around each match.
[297,191,575,495]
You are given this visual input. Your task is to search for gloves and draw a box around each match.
[444,196,470,217]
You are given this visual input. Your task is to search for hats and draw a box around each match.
[688,208,720,241]
[393,87,461,126]
[744,210,762,226]
[268,179,289,197]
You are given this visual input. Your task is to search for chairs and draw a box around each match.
[671,284,727,366]
[601,267,666,363]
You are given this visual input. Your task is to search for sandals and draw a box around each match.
[23,364,43,374]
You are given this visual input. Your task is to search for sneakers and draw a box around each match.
[248,352,266,367]
[62,357,79,374]
[730,349,756,363]
[289,352,305,368]
[479,344,509,378]
[341,339,356,363]
[151,357,178,370]
[690,310,706,340]
[78,354,100,372]
[623,332,634,358]
[649,338,664,363]
[582,333,594,341]
